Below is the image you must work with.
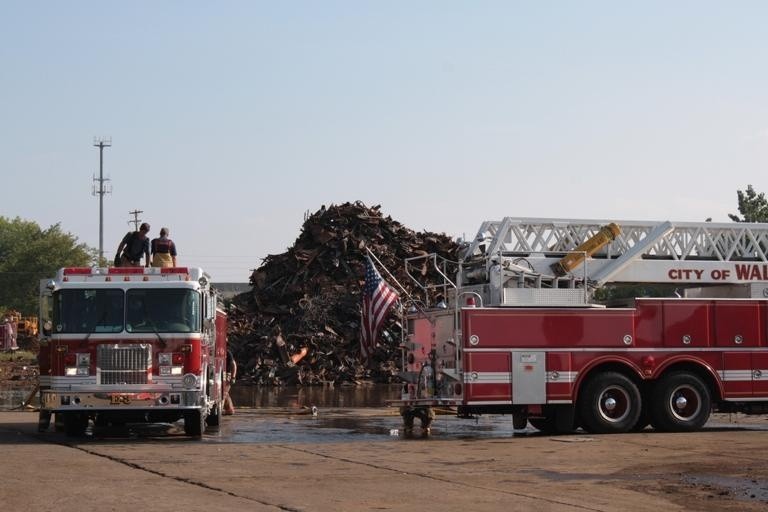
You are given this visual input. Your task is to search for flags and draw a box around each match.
[359,255,399,369]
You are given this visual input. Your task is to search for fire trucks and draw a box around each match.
[397,215,768,437]
[35,265,231,441]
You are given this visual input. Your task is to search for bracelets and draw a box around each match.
[231,377,235,379]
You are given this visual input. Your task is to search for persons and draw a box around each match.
[151,228,177,267]
[223,349,237,415]
[400,406,435,432]
[10,318,17,347]
[4,319,13,347]
[115,223,151,267]
